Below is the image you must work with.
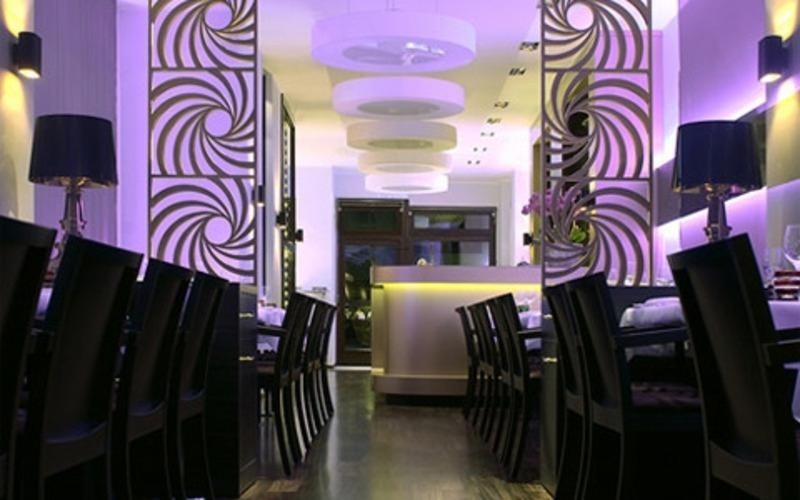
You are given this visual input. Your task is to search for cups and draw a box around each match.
[781,223,800,272]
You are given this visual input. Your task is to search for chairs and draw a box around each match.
[0,215,57,499]
[16,233,142,499]
[169,270,230,499]
[564,272,707,499]
[112,255,192,500]
[256,290,338,476]
[540,279,587,500]
[666,232,800,499]
[454,295,542,482]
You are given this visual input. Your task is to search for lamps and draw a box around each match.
[671,119,763,243]
[757,35,783,86]
[30,111,117,289]
[11,33,42,82]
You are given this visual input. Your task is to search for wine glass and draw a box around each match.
[513,291,537,313]
